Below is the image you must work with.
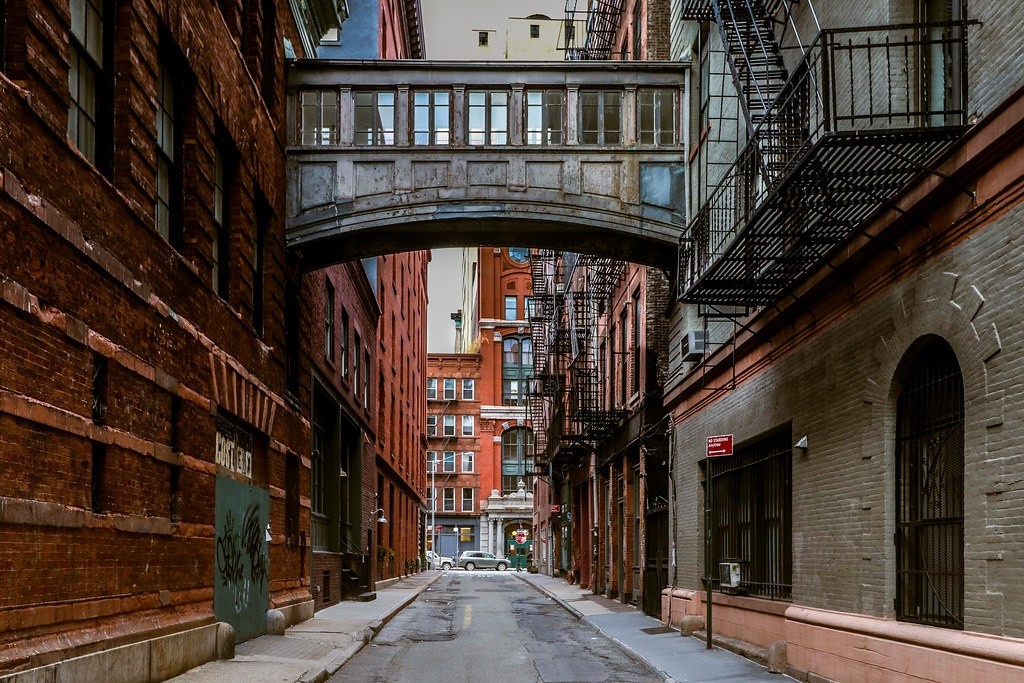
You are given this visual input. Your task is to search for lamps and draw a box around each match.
[369,509,388,524]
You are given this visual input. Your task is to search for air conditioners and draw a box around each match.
[534,382,543,393]
[719,563,741,587]
[680,330,709,362]
[556,283,564,293]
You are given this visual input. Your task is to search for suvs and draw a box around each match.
[457,550,513,572]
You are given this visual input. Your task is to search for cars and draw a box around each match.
[426,551,456,570]
